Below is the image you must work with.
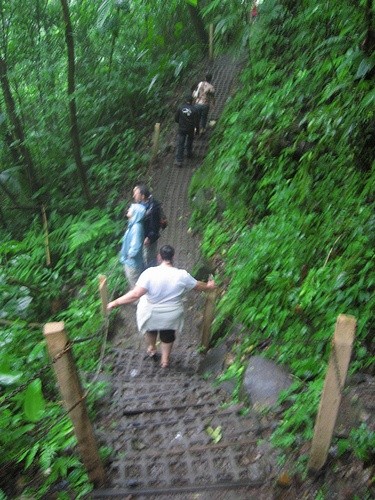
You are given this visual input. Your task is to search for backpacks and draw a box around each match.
[148,200,169,231]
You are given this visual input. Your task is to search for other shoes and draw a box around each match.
[158,361,170,372]
[186,150,196,158]
[199,128,206,134]
[194,127,199,134]
[173,160,183,167]
[143,344,157,361]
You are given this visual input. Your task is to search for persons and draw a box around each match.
[192,74,217,134]
[250,5,258,21]
[119,204,147,305]
[133,185,160,267]
[175,96,198,167]
[107,245,215,368]
